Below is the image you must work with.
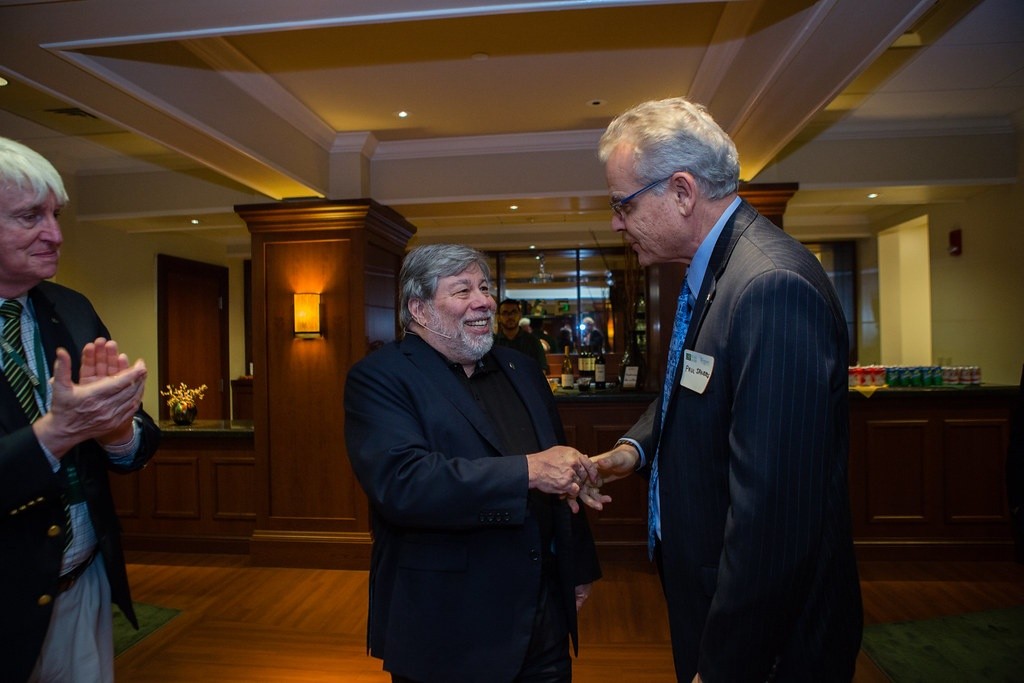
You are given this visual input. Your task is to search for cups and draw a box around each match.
[576,377,592,391]
[548,378,559,394]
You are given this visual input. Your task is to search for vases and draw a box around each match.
[170,398,197,425]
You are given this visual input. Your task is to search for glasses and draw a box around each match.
[608,172,675,221]
[498,310,518,316]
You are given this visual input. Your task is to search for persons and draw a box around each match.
[521,317,605,353]
[496,300,550,377]
[0,136,165,683]
[343,245,600,683]
[559,96,864,683]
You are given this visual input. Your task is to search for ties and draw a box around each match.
[0,299,74,554]
[645,268,692,563]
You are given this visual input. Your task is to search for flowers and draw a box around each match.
[160,382,208,408]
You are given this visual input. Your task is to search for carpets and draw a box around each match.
[860,603,1024,683]
[112,600,181,659]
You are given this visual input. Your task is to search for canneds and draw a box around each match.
[848,365,982,386]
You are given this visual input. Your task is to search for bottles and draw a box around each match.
[595,345,606,390]
[577,344,597,377]
[618,329,645,394]
[560,346,574,390]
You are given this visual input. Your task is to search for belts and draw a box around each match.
[55,546,100,596]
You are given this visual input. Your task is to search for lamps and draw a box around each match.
[293,292,322,337]
